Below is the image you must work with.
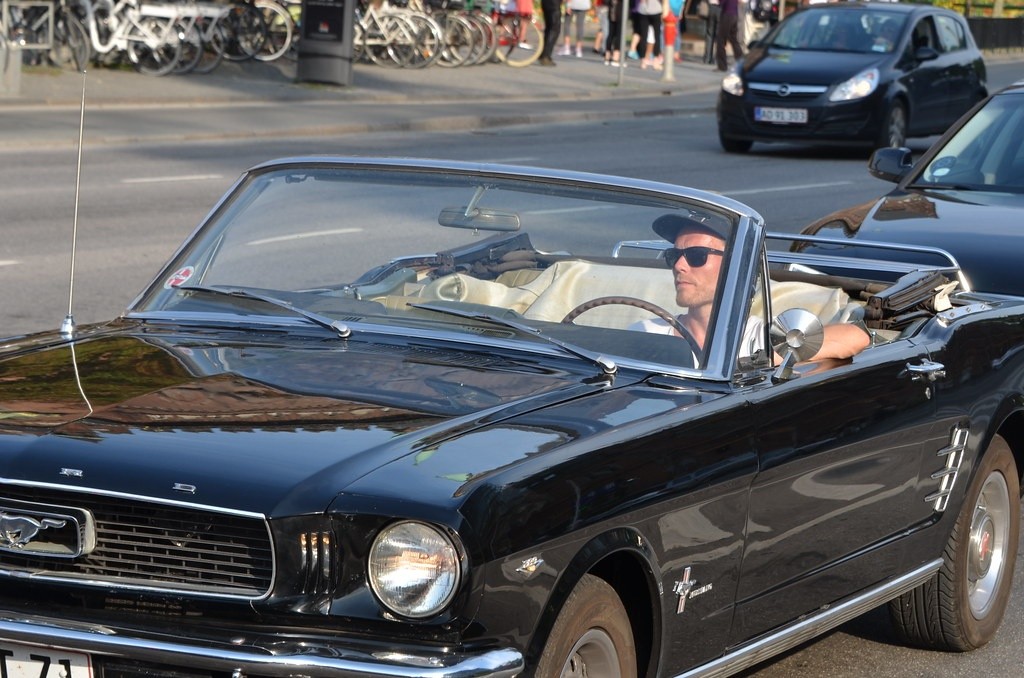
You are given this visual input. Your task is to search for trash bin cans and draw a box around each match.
[295,0,356,89]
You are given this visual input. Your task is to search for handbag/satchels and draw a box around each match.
[636,0,663,15]
[697,0,710,18]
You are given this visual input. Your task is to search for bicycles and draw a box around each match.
[0,0,546,78]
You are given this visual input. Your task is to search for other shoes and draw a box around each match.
[627,51,639,61]
[540,57,556,66]
[576,49,583,58]
[519,42,533,50]
[604,60,627,68]
[559,46,572,57]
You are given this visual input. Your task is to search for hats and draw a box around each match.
[653,209,736,244]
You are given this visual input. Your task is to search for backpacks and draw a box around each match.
[751,0,772,22]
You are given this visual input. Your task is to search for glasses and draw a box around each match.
[663,245,724,267]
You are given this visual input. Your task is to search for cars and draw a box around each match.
[716,5,987,157]
[0,154,1024,678]
[774,83,1024,296]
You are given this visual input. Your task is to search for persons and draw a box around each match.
[592,0,691,71]
[830,23,855,51]
[697,0,743,72]
[628,212,871,371]
[491,0,535,50]
[538,0,563,66]
[742,0,773,56]
[871,20,913,53]
[557,0,592,58]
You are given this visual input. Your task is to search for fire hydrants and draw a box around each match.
[661,12,678,80]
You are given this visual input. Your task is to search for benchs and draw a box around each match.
[442,263,862,343]
